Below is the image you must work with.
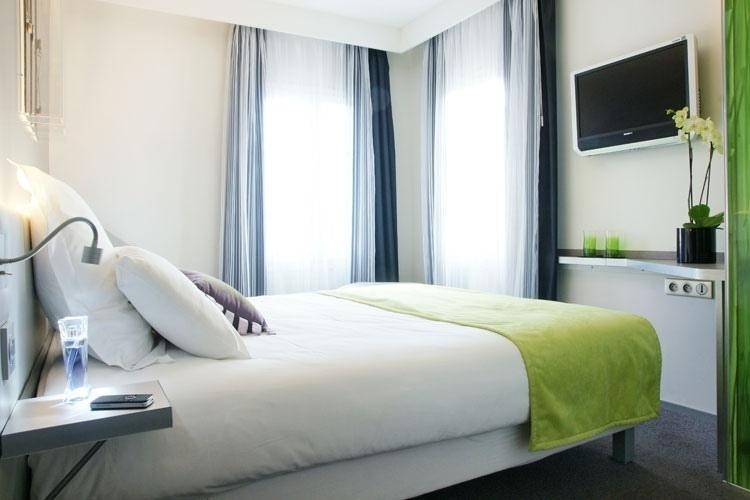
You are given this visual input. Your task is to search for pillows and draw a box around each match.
[9,158,175,371]
[181,268,276,336]
[114,245,250,358]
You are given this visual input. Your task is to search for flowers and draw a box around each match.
[668,107,721,227]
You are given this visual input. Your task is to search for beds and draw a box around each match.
[0,208,661,498]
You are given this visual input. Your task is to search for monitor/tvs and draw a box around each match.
[572,31,702,157]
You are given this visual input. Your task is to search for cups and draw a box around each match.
[583,230,596,255]
[57,316,91,402]
[605,231,620,258]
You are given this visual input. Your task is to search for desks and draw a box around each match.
[2,381,173,499]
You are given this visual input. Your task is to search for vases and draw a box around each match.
[675,228,716,263]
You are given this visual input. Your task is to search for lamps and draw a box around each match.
[0,215,102,276]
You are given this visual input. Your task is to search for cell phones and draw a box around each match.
[91,392,155,411]
